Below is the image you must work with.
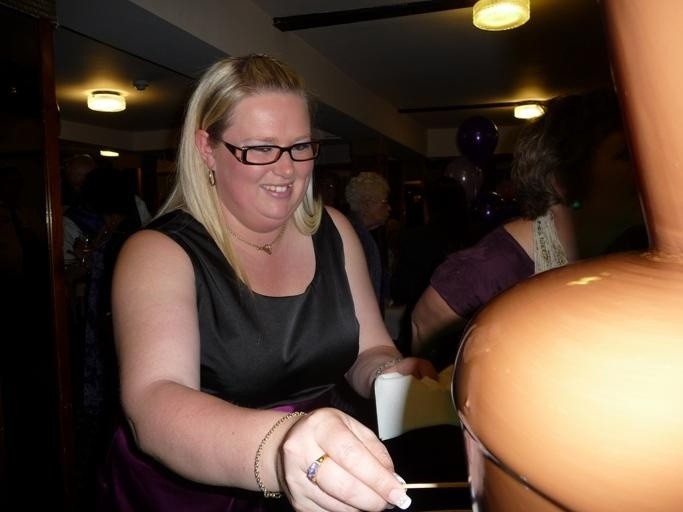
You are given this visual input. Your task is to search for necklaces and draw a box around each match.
[219,216,292,257]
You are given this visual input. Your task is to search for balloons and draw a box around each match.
[438,117,520,219]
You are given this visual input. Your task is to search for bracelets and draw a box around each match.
[373,356,402,377]
[251,408,310,499]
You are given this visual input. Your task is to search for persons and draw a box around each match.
[406,92,640,367]
[62,154,154,280]
[107,53,440,512]
[340,170,393,302]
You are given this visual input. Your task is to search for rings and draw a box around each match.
[304,452,330,486]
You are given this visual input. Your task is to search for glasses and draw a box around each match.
[220,138,321,165]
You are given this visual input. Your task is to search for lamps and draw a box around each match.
[99,149,119,157]
[85,90,126,113]
[514,104,545,120]
[471,0,531,31]
